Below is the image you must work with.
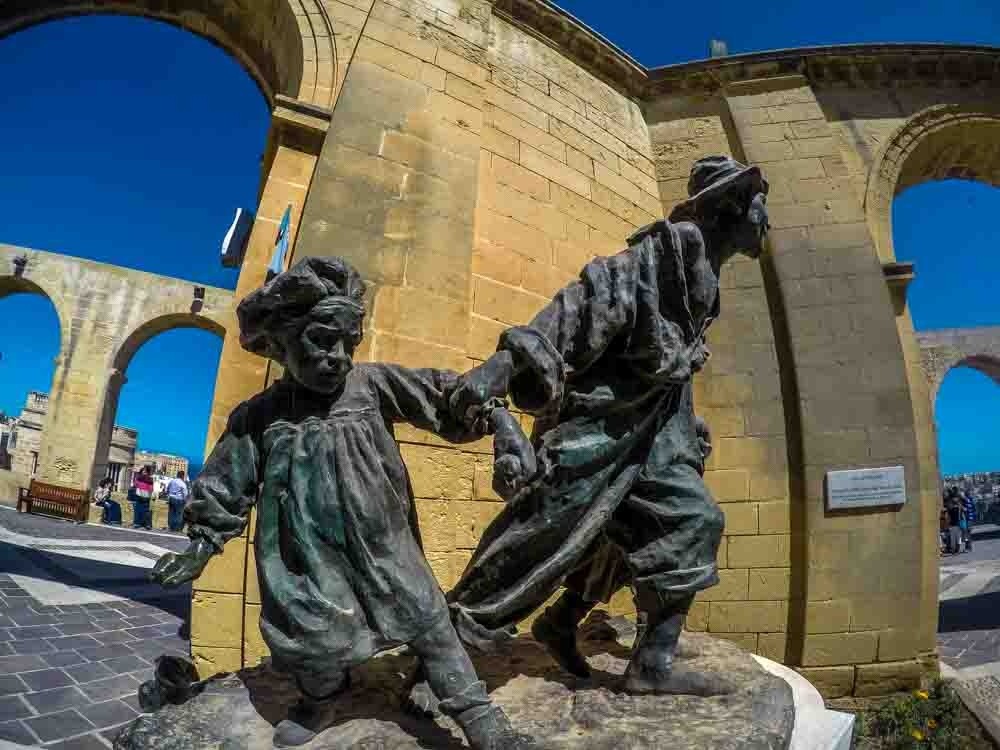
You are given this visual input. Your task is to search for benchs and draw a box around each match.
[16,478,93,525]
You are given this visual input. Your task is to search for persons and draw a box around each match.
[166,471,188,532]
[941,486,978,556]
[404,155,774,715]
[151,256,538,750]
[94,477,122,525]
[133,465,154,531]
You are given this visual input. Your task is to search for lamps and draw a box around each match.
[193,285,206,302]
[14,255,28,267]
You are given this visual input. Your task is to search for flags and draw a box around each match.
[266,203,292,283]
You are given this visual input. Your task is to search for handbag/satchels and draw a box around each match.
[127,487,139,501]
